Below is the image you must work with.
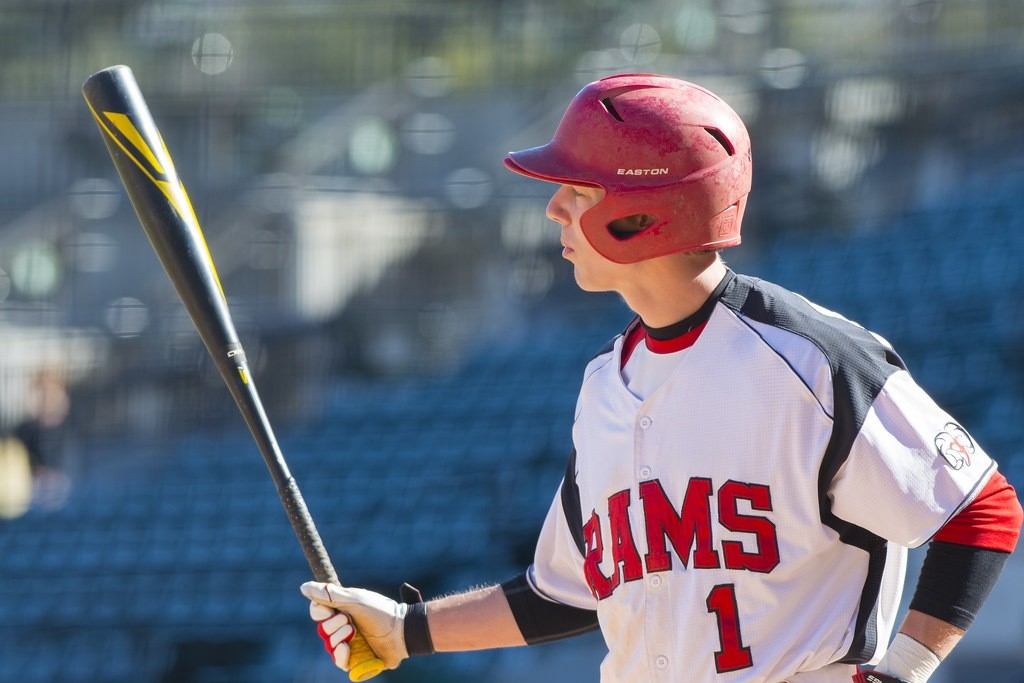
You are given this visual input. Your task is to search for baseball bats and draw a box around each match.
[79,63,387,683]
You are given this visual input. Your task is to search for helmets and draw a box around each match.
[504,73,753,265]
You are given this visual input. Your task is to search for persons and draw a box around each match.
[300,75,1023,683]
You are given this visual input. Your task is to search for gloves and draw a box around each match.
[300,581,434,670]
